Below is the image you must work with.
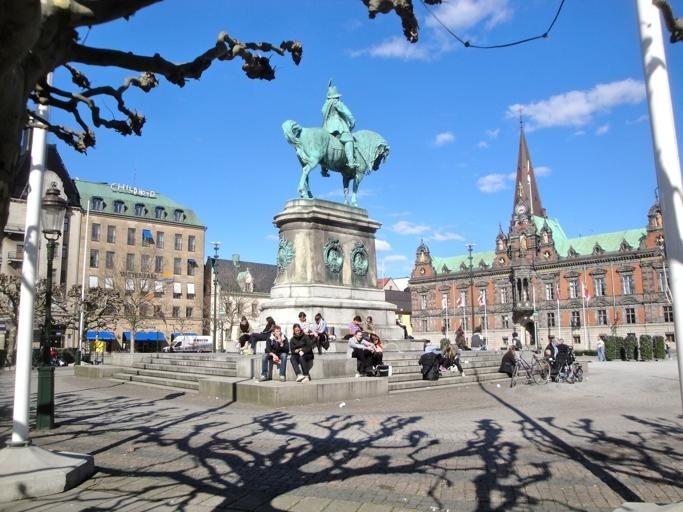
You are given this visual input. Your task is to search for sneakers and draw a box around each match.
[236,341,254,355]
[260,375,310,383]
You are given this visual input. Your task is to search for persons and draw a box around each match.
[665,338,673,359]
[444,346,467,377]
[319,78,361,178]
[236,309,384,382]
[498,331,565,384]
[596,336,607,361]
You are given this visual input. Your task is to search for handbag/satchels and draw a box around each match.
[376,365,389,376]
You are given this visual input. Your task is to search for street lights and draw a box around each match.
[34,179,67,432]
[211,239,221,354]
[465,243,476,333]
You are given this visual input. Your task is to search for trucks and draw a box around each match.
[163,334,211,354]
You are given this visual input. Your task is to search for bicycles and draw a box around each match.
[510,346,584,387]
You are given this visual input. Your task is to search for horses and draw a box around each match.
[281,119,392,209]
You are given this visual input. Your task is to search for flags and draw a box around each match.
[581,282,591,304]
[477,292,485,306]
[456,293,465,308]
[556,288,561,301]
[442,298,447,309]
